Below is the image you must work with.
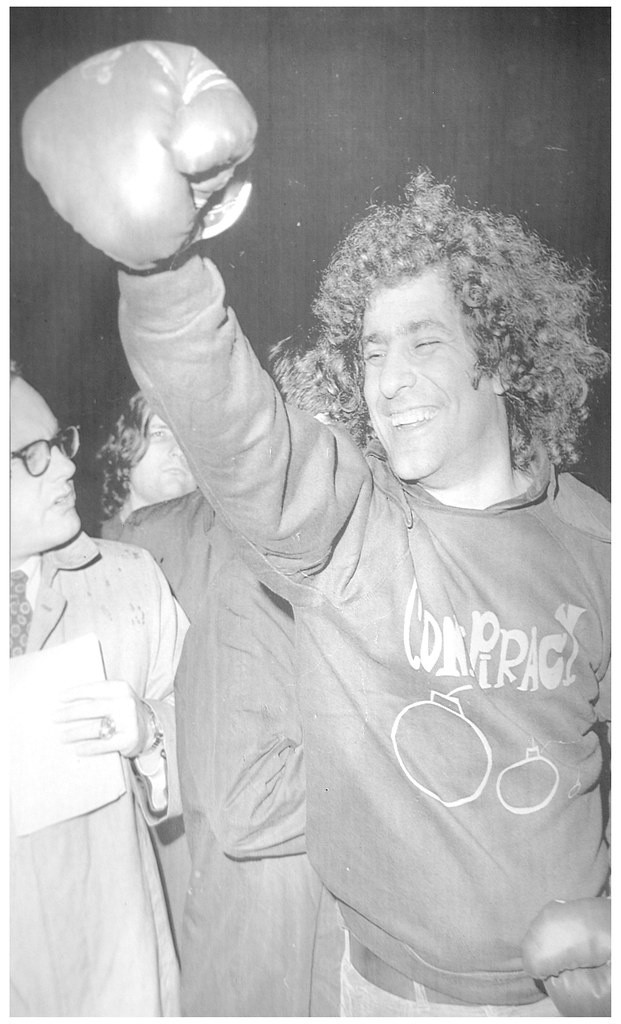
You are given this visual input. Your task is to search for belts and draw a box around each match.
[348,933,486,1007]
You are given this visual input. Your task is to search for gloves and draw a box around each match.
[19,41,258,270]
[520,898,610,1017]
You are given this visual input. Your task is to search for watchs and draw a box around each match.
[136,701,164,757]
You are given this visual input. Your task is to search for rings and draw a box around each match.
[99,715,116,740]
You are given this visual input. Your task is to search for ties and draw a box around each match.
[9,571,32,658]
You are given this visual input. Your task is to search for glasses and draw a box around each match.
[10,426,80,478]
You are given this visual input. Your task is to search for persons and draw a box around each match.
[21,40,611,1016]
[97,328,375,1017]
[9,360,191,1016]
